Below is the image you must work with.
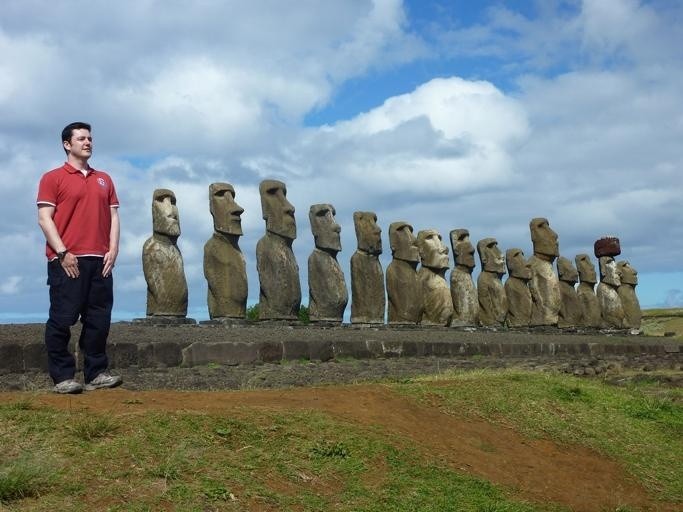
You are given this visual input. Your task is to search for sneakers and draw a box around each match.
[53,380,83,394]
[86,373,122,391]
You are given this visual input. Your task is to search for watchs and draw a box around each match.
[56,250,67,259]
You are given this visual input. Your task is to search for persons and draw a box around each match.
[307,204,348,322]
[35,122,121,393]
[386,218,642,330]
[203,183,248,318]
[256,180,302,320]
[142,188,188,316]
[351,211,386,324]
